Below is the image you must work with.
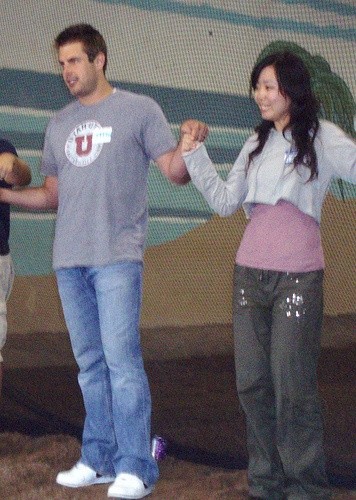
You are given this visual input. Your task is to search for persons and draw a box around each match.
[0,23,209,500]
[0,139,33,365]
[182,52,356,499]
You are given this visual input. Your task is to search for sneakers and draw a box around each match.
[55,461,116,488]
[107,473,153,499]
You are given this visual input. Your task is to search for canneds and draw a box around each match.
[151,435,168,462]
